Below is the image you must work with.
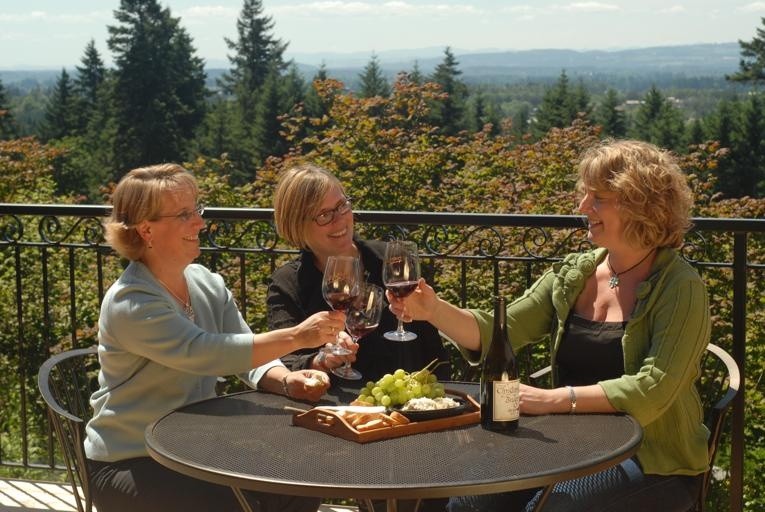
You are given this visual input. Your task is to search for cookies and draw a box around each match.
[333,408,410,431]
[303,374,325,393]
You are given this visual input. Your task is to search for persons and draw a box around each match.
[386,137,710,511]
[265,162,451,382]
[83,162,322,512]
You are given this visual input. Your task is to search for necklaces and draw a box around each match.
[607,248,656,289]
[156,277,192,317]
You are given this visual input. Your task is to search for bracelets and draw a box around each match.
[280,372,290,399]
[567,385,577,413]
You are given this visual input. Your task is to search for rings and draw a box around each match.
[331,326,335,335]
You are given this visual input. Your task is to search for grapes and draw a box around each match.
[358,367,446,405]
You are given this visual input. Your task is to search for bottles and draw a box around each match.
[479,296,521,432]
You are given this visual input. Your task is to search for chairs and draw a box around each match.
[689,342,741,511]
[37,346,103,512]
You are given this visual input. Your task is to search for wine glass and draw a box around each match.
[328,282,383,381]
[318,255,367,358]
[380,239,421,344]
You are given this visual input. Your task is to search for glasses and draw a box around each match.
[152,203,208,225]
[304,196,355,226]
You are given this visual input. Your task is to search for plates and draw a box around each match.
[387,392,471,423]
